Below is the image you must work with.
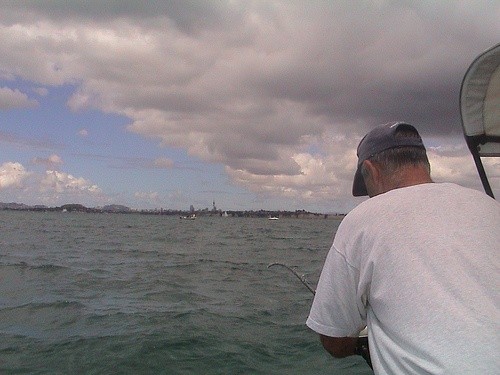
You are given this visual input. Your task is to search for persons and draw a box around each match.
[304,119,499,375]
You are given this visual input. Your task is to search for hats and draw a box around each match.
[352,120,423,196]
